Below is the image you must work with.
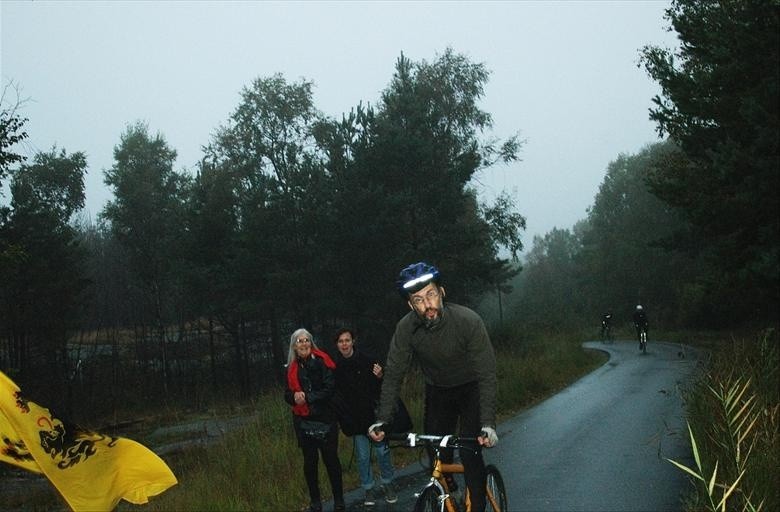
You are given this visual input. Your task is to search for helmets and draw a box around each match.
[397,261,440,295]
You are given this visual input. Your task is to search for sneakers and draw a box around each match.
[384,484,398,503]
[415,477,457,498]
[364,491,376,505]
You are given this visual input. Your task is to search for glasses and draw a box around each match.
[296,338,311,343]
[411,290,438,307]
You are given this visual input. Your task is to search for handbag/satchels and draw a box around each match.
[327,390,353,420]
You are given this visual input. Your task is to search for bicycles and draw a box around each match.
[598,323,620,348]
[366,422,511,511]
[634,323,651,353]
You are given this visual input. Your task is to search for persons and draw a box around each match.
[602,307,616,345]
[633,305,648,350]
[367,263,499,512]
[333,327,398,506]
[283,328,345,512]
